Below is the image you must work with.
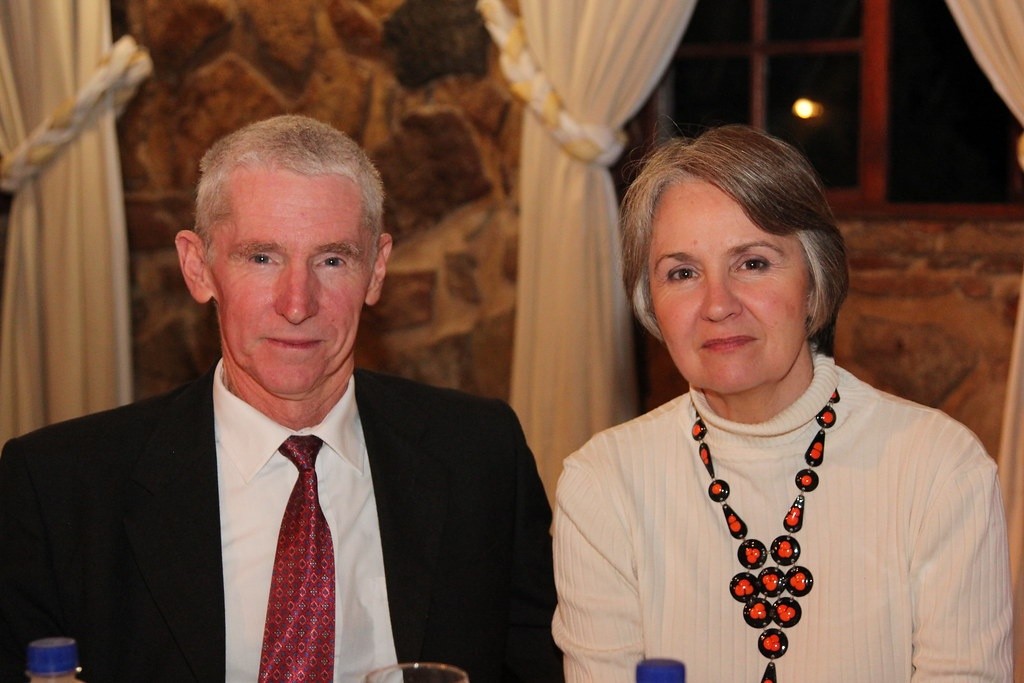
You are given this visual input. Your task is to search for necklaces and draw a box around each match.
[692,386,839,683]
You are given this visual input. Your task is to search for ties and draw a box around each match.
[258,435,335,683]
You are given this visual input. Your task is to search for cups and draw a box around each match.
[365,661,468,683]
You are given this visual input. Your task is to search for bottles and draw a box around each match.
[25,636,84,683]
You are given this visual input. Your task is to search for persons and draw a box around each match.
[0,116,566,683]
[552,124,1012,682]
